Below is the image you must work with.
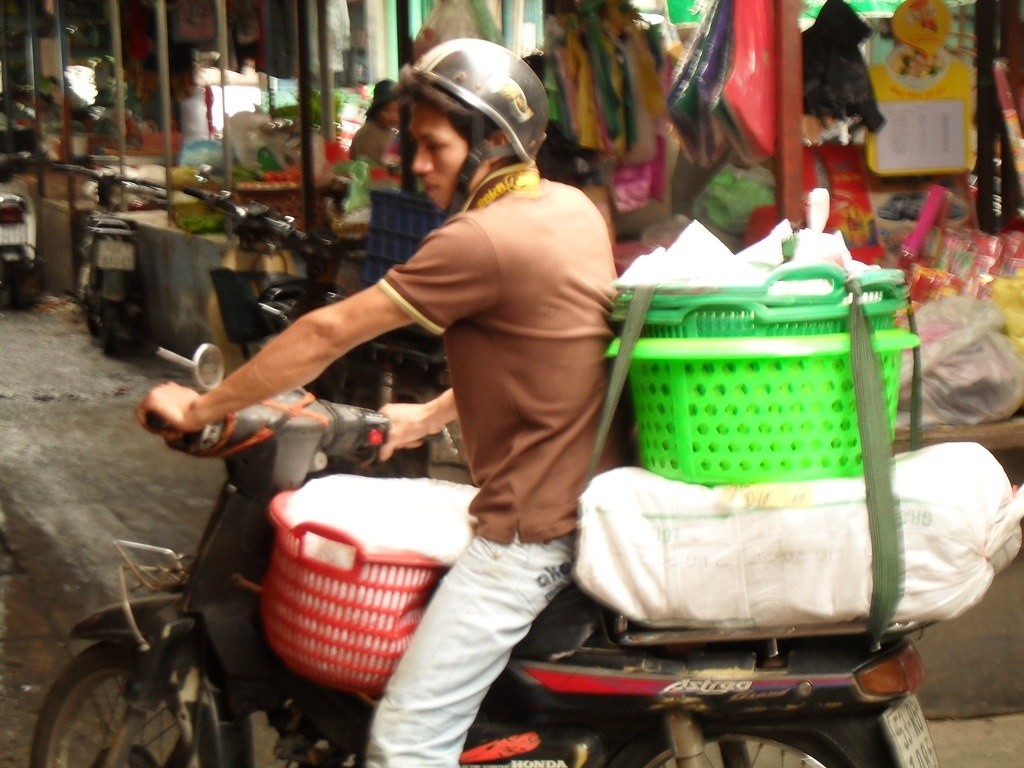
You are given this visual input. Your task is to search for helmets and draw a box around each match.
[397,38,547,167]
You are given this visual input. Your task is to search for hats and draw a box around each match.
[365,79,396,116]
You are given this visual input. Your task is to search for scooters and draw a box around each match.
[182,162,431,576]
[0,97,43,309]
[48,161,169,358]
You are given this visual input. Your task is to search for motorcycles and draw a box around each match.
[30,344,942,768]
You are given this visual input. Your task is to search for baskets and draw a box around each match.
[256,468,480,691]
[610,267,913,337]
[614,329,923,481]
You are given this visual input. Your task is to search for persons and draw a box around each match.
[350,79,399,166]
[134,37,618,768]
